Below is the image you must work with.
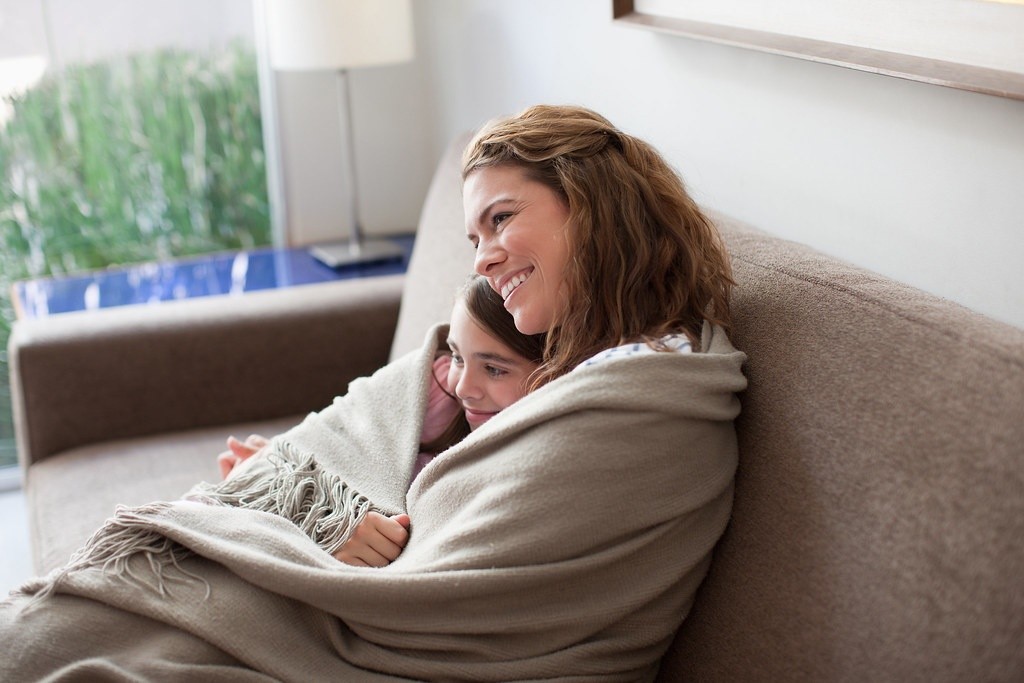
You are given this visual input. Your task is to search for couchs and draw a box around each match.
[8,133,1024,683]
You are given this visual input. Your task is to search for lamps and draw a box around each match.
[259,0,414,269]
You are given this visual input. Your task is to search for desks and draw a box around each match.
[12,232,414,317]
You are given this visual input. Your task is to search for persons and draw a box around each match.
[0,105,747,683]
